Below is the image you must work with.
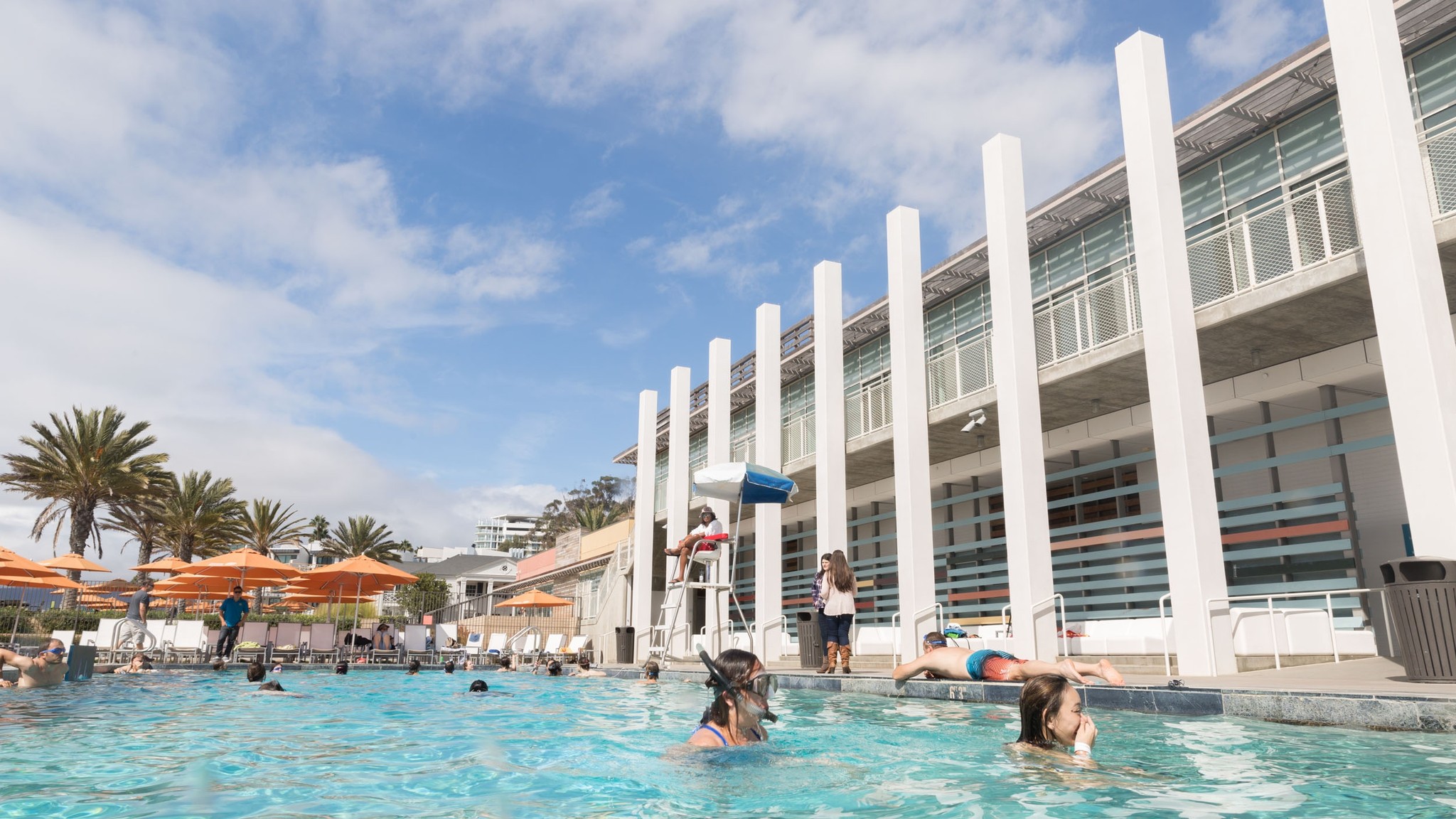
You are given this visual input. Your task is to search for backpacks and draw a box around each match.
[343,632,372,652]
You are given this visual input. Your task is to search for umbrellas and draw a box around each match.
[1,546,418,663]
[693,461,800,594]
[494,589,575,635]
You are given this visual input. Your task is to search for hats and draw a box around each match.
[698,507,716,518]
[379,625,390,629]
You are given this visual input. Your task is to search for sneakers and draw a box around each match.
[209,656,233,663]
[141,655,155,661]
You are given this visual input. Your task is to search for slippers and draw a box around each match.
[668,578,684,583]
[663,548,681,557]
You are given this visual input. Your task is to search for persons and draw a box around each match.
[237,679,314,699]
[213,659,227,671]
[533,659,554,676]
[812,553,832,674]
[129,654,151,674]
[405,659,421,675]
[0,638,69,690]
[661,649,866,778]
[634,660,691,685]
[454,680,514,699]
[574,657,607,678]
[1003,672,1181,792]
[549,661,575,678]
[236,663,266,686]
[821,550,857,674]
[663,506,722,583]
[463,659,474,671]
[330,661,348,676]
[443,661,455,674]
[893,631,1124,689]
[270,663,283,674]
[496,654,517,673]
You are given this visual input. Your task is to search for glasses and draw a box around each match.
[731,673,778,696]
[273,667,285,670]
[702,513,710,518]
[149,583,154,587]
[39,648,65,655]
[381,628,388,630]
[234,591,242,593]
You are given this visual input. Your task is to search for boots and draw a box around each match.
[817,655,830,673]
[839,644,851,674]
[826,640,838,673]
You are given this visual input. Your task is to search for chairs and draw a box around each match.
[685,541,723,584]
[0,629,589,669]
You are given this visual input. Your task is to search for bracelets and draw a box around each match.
[1073,742,1091,753]
